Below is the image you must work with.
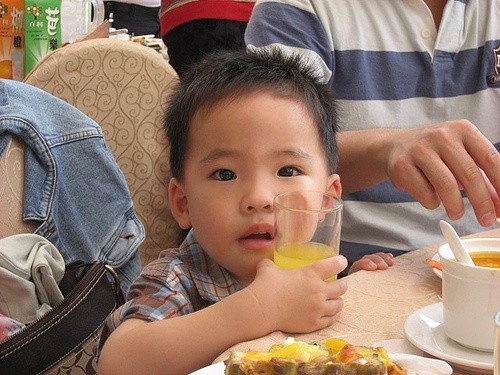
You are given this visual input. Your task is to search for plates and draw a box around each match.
[432,252,442,278]
[186,352,454,374]
[404,301,495,374]
[108,29,161,51]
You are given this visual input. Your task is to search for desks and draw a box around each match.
[217,229,500,374]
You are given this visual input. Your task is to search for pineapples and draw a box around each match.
[223,338,387,375]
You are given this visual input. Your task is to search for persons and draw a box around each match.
[91,0,256,71]
[95,49,395,375]
[245,0,500,278]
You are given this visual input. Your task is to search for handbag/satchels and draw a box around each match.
[0,261,126,375]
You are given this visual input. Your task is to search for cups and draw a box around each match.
[437,237,500,351]
[273,190,343,282]
[491,313,500,375]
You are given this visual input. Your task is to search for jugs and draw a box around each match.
[60,0,105,44]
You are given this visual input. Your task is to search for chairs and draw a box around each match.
[30,38,187,255]
[1,72,152,375]
